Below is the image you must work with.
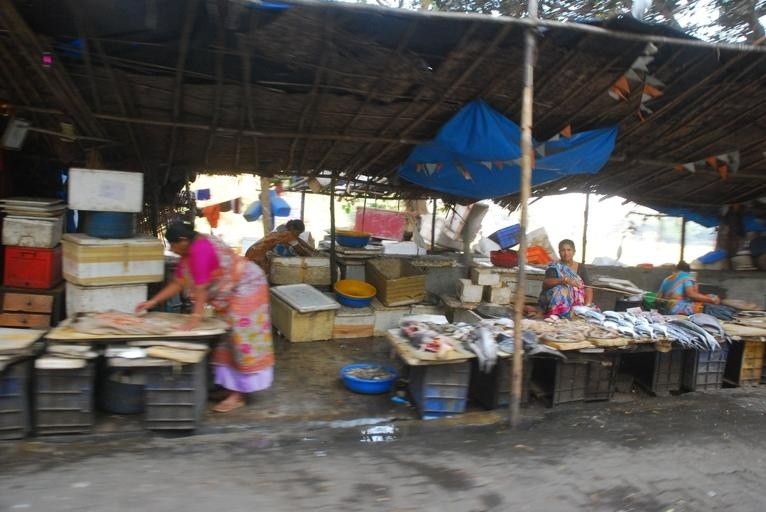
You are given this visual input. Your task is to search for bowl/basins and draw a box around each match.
[339,364,400,395]
[643,292,659,310]
[331,280,376,308]
[335,230,372,248]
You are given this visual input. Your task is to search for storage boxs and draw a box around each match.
[269,248,547,346]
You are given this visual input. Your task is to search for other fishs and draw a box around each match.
[400,305,733,371]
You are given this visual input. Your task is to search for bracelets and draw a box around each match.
[191,313,201,318]
[149,298,159,306]
[562,277,568,283]
[586,304,592,307]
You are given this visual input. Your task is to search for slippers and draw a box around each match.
[212,401,245,412]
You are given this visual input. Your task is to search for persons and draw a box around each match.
[656,262,719,315]
[747,231,766,271]
[245,219,320,277]
[135,221,276,414]
[540,238,593,320]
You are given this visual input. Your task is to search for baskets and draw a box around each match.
[487,223,554,268]
